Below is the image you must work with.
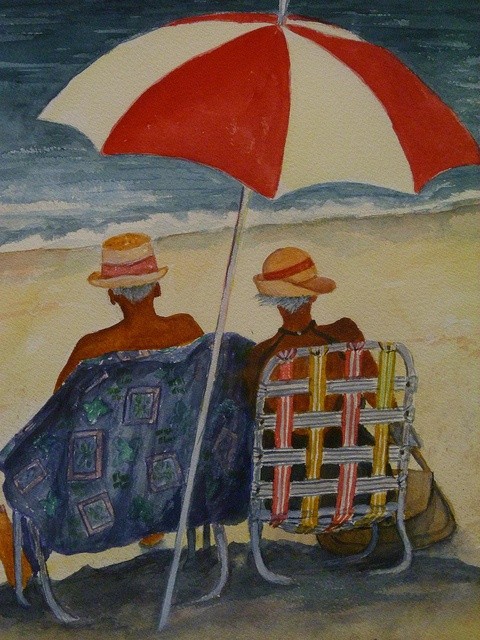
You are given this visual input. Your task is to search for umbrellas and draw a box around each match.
[36,0,479,634]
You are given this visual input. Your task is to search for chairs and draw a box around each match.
[2,329,244,627]
[203,340,423,586]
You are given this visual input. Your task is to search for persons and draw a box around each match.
[243,246,402,511]
[0,233,206,592]
